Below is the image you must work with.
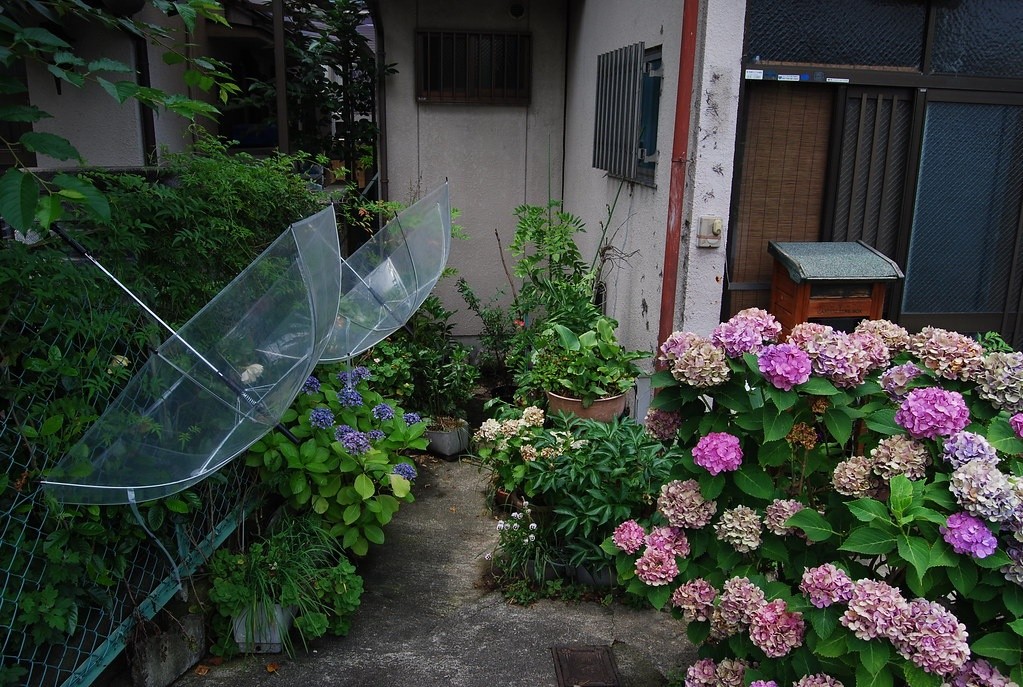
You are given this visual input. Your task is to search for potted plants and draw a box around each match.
[417,343,477,459]
[206,519,363,656]
[514,318,654,425]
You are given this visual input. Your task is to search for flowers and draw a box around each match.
[473,408,590,488]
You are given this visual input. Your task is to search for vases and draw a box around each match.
[489,477,556,522]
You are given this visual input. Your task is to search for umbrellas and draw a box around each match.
[252,176,451,358]
[35,197,341,504]
